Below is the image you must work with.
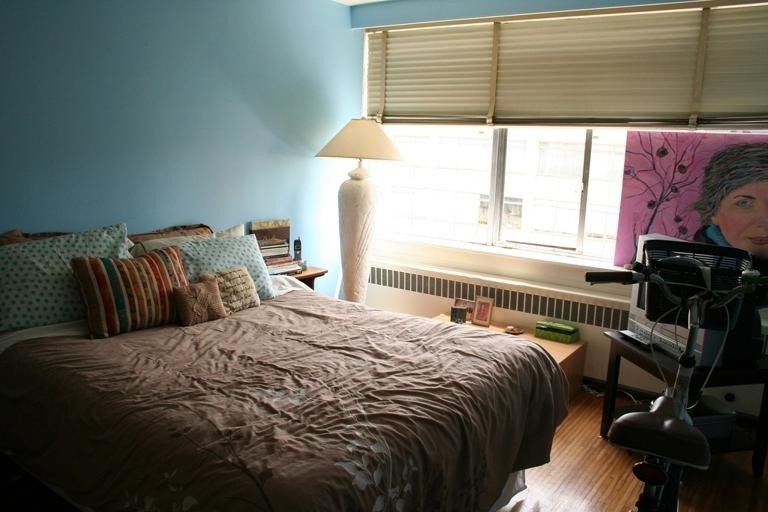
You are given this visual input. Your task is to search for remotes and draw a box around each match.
[619,328,654,348]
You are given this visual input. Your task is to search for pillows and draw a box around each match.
[149,232,275,300]
[69,246,189,339]
[135,223,245,256]
[172,277,227,326]
[0,222,134,334]
[200,265,260,315]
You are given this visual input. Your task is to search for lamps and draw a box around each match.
[314,118,398,303]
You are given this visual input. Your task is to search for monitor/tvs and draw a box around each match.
[628,232,763,369]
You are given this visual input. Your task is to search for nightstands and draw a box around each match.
[291,265,328,290]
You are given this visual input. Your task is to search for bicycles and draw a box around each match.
[585,261,768,512]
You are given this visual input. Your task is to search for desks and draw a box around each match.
[431,312,589,404]
[600,328,768,479]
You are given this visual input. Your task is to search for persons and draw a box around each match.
[476,302,488,321]
[690,141,767,259]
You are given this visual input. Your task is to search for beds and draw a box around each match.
[0,223,570,511]
